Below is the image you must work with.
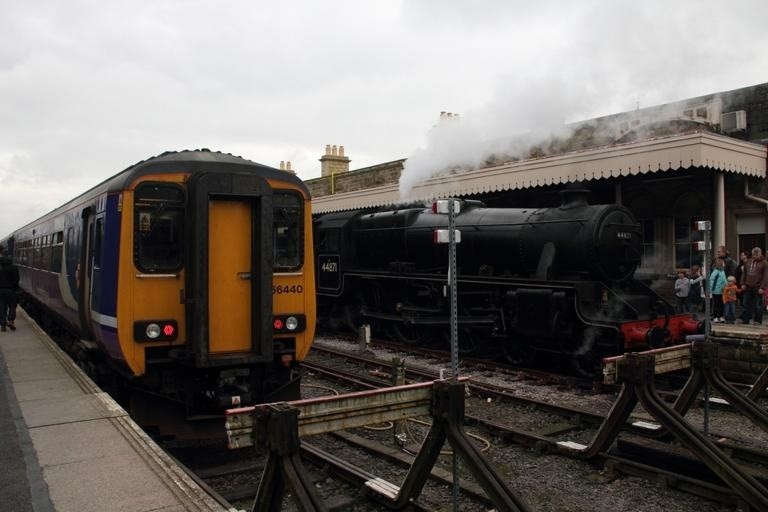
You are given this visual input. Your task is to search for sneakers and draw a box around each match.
[7,320,16,330]
[714,317,763,325]
[1,325,7,332]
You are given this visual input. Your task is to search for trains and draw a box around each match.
[0,147,318,458]
[310,195,706,393]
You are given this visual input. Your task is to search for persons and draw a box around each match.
[0,251,21,333]
[672,247,768,324]
